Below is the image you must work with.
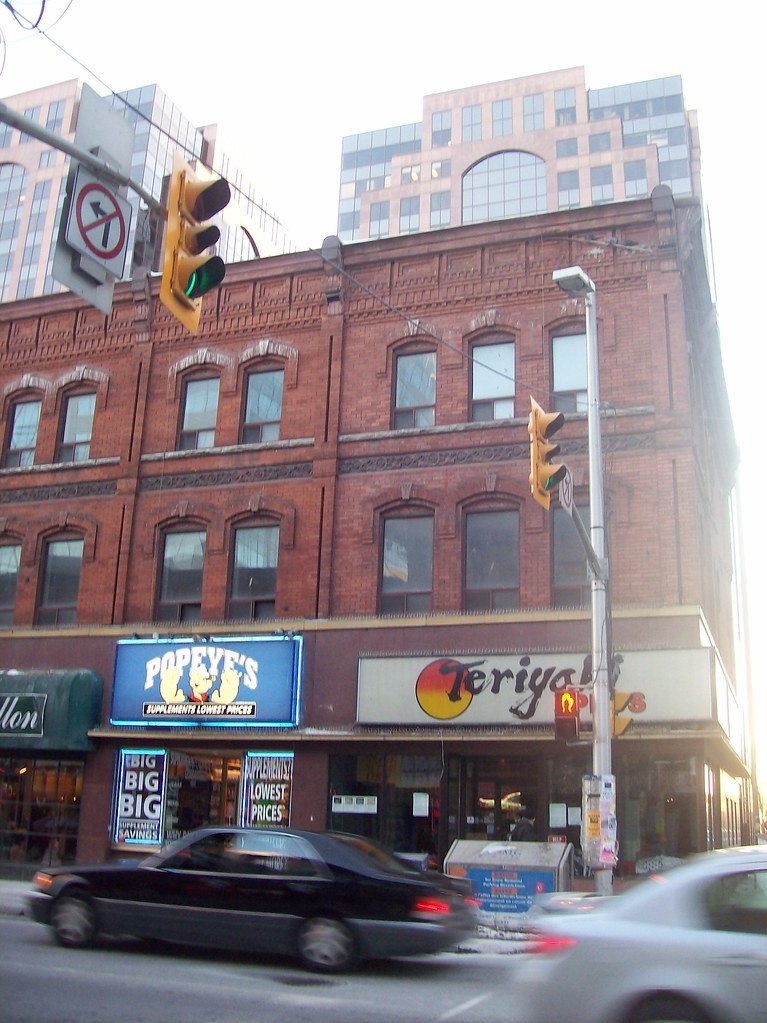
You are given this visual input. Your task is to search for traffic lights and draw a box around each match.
[531,400,567,508]
[554,689,579,744]
[609,691,632,740]
[159,147,231,335]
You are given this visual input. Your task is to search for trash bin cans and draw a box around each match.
[443,838,574,935]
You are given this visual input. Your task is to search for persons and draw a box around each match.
[510,805,537,841]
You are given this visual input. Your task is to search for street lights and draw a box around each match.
[554,265,613,898]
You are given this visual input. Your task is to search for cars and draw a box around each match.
[513,842,766,1022]
[25,824,478,971]
